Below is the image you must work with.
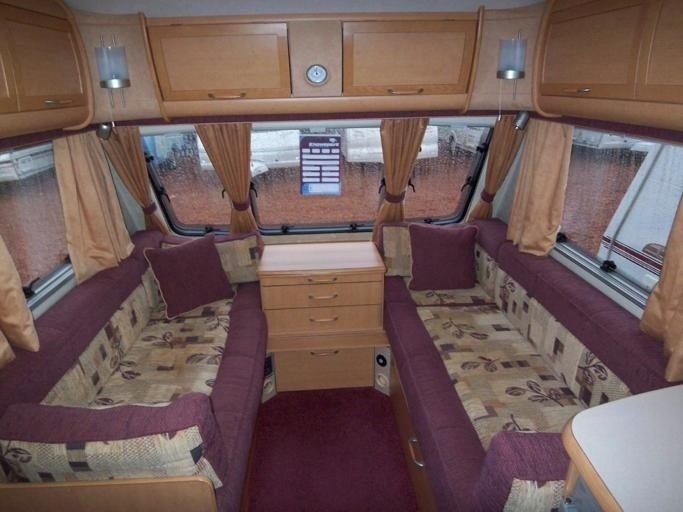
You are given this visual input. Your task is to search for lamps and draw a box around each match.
[94,32,131,109]
[497,28,527,99]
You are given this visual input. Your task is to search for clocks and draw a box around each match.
[304,64,329,86]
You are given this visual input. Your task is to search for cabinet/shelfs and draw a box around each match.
[342,19,478,97]
[255,241,391,393]
[635,0,683,104]
[0,0,89,112]
[145,22,292,101]
[540,0,642,101]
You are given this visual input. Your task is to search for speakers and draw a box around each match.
[373,346,403,398]
[260,352,278,405]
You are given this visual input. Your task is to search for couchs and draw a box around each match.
[0,227,269,512]
[380,216,673,512]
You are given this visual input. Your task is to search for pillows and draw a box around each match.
[408,222,480,291]
[143,232,235,320]
